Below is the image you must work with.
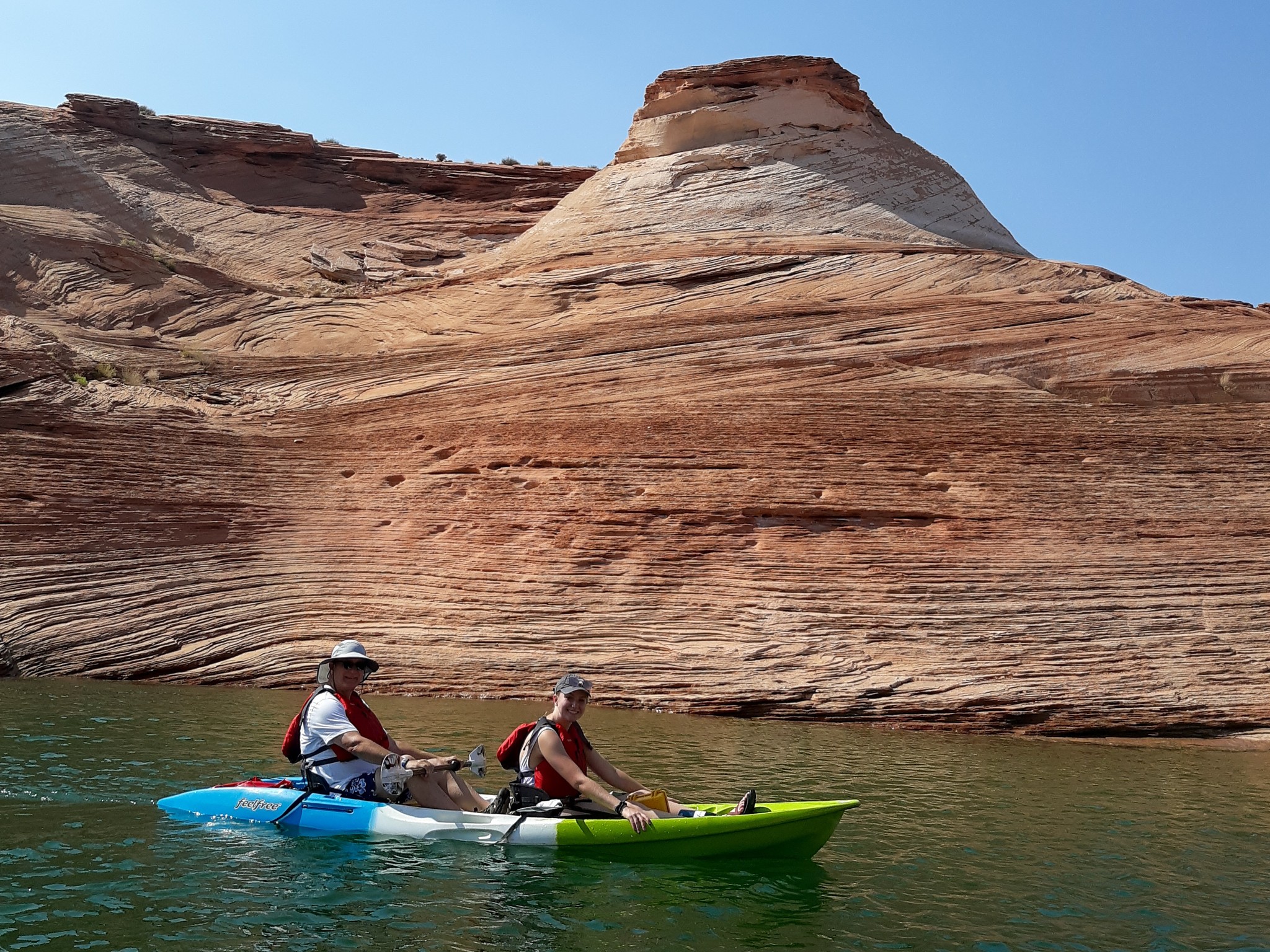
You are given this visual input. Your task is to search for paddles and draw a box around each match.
[379,744,487,796]
[515,791,627,812]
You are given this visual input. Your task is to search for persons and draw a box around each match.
[300,639,510,814]
[517,674,756,835]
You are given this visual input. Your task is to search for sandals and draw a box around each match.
[726,793,750,816]
[748,789,756,814]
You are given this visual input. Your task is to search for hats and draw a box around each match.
[316,640,380,684]
[553,673,591,698]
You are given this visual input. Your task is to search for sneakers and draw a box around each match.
[473,787,510,813]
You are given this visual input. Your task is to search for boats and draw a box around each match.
[156,775,862,863]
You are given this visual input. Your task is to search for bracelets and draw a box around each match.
[615,797,630,816]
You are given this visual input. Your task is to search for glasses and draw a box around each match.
[556,677,592,692]
[334,659,365,671]
